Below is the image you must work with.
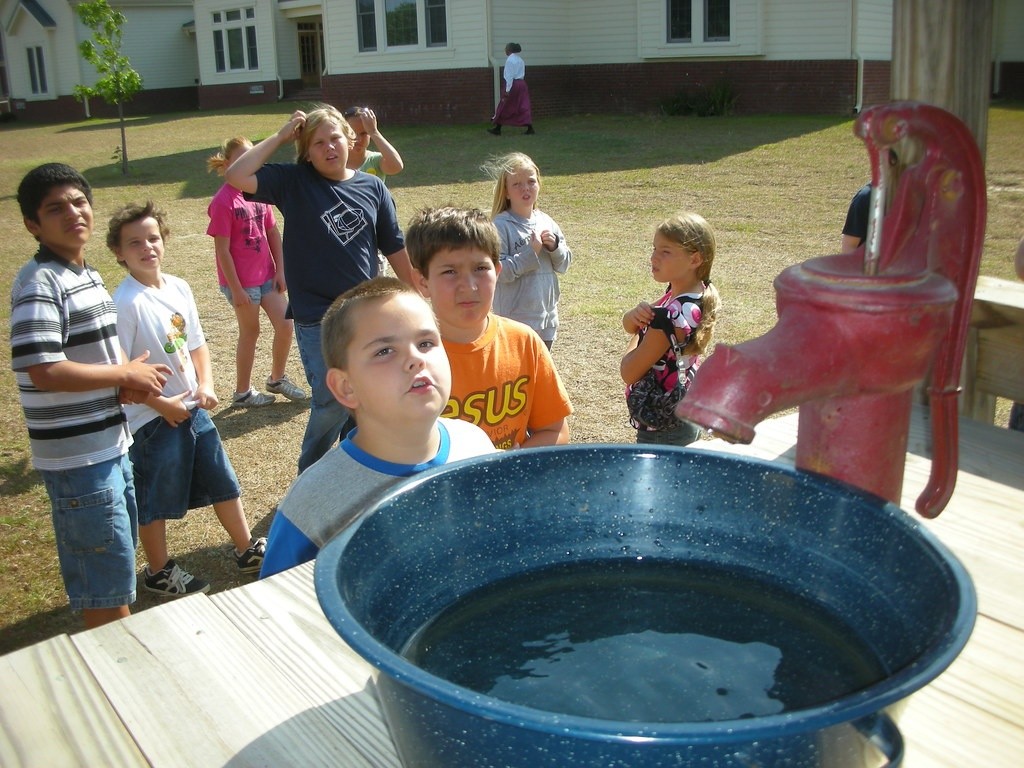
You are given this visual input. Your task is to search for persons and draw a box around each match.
[487,41,536,137]
[342,107,404,278]
[619,211,720,448]
[257,276,497,582]
[1006,235,1024,431]
[485,152,572,353]
[404,205,575,455]
[840,180,874,255]
[221,106,422,478]
[207,135,307,406]
[9,163,174,631]
[104,201,267,598]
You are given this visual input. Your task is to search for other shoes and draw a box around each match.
[488,128,501,135]
[524,128,535,135]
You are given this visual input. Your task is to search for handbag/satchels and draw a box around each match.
[627,308,688,432]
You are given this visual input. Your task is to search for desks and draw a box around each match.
[0,402,1022,768]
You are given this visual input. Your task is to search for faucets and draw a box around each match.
[675,98,987,519]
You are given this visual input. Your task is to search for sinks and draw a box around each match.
[314,443,978,768]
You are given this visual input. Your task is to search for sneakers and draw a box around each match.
[233,537,268,573]
[233,386,276,407]
[266,374,306,401]
[145,559,211,597]
